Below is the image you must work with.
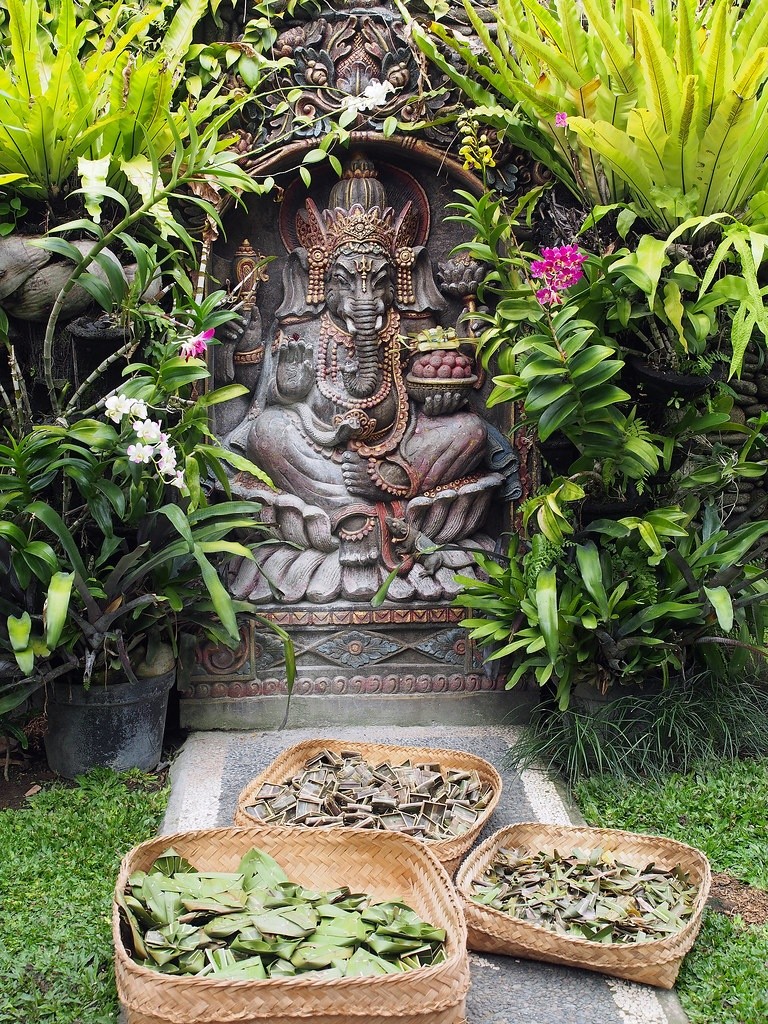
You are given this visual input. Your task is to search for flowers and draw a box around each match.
[0,81,396,730]
[367,244,768,713]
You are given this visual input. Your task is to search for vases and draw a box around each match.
[38,668,178,777]
[573,671,681,758]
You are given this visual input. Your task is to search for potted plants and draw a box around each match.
[2,0,283,433]
[398,1,768,483]
